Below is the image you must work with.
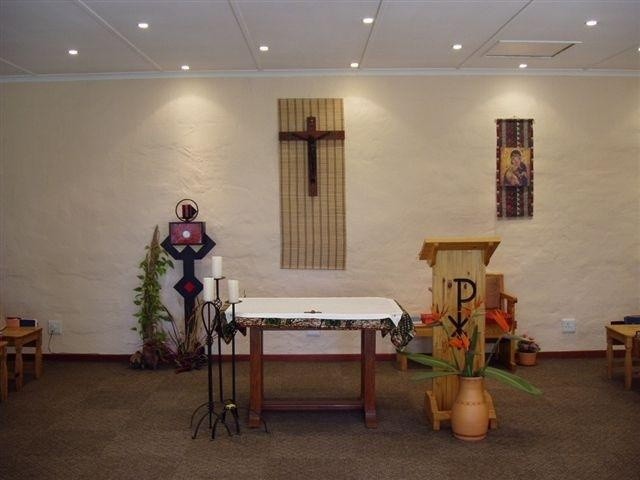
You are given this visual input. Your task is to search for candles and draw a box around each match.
[202,255,241,304]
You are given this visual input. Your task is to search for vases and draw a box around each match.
[449,372,490,441]
[519,353,538,366]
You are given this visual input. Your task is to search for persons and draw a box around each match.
[501,148,528,187]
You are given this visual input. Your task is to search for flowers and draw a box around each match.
[516,332,540,352]
[397,294,544,398]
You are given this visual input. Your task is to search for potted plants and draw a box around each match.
[129,224,174,369]
[156,296,220,374]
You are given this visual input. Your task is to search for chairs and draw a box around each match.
[485,272,518,375]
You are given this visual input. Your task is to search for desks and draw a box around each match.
[222,297,408,430]
